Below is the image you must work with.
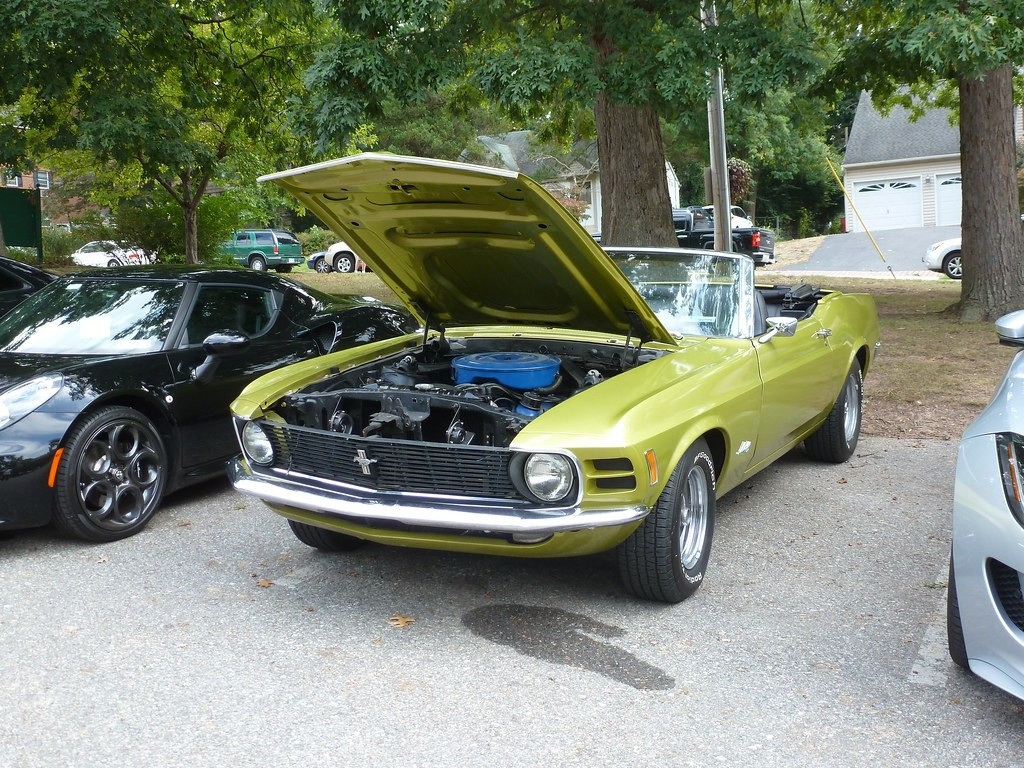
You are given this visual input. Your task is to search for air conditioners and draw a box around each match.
[40,190,49,198]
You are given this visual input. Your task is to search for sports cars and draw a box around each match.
[226,149,886,604]
[0,264,421,543]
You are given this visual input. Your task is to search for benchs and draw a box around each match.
[642,285,793,337]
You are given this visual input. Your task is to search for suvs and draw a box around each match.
[671,205,712,225]
[701,204,753,231]
[218,229,305,277]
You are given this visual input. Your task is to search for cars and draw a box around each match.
[0,254,74,319]
[945,304,1024,703]
[305,252,335,274]
[70,238,160,268]
[325,241,375,273]
[922,214,1024,284]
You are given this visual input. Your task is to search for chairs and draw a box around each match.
[691,284,769,339]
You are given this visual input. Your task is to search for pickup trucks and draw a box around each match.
[659,213,776,272]
